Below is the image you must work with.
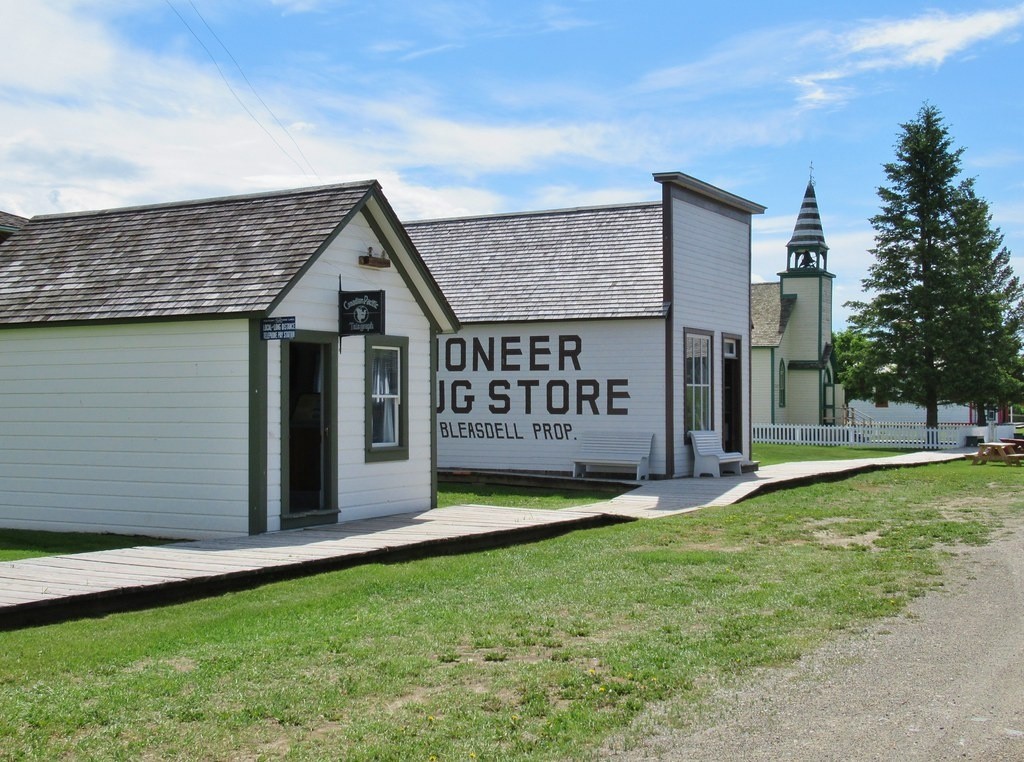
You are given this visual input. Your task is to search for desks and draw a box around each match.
[972,443,1021,467]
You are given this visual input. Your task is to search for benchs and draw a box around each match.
[570,431,655,481]
[965,453,985,460]
[687,431,744,479]
[1006,453,1024,460]
